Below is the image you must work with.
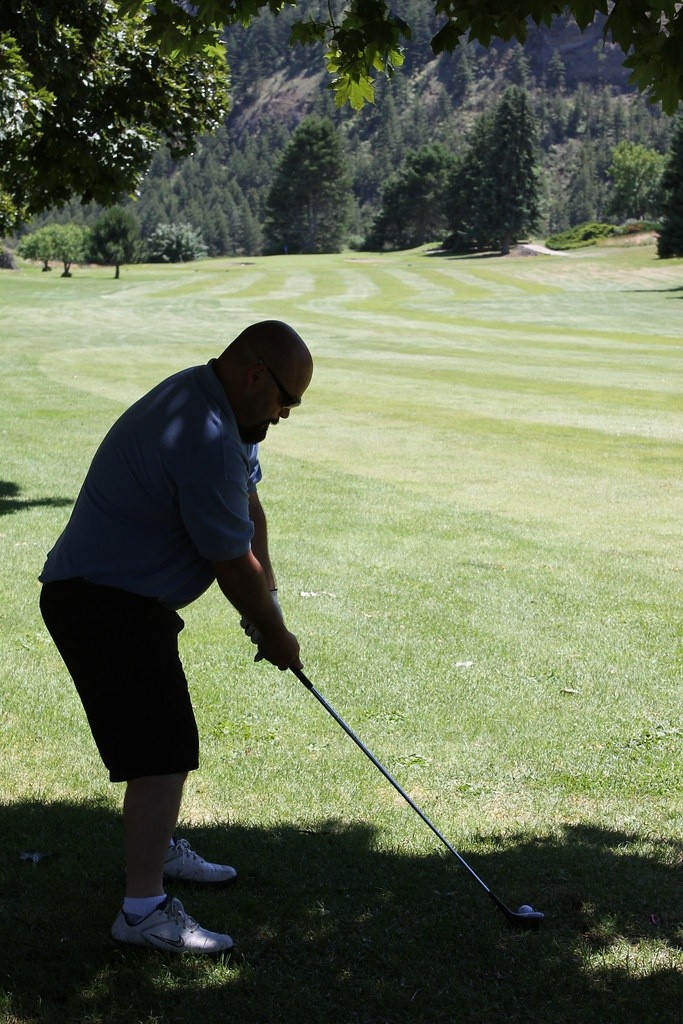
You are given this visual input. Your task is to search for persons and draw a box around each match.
[38,320,313,955]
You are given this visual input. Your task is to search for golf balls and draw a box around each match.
[519,903,534,914]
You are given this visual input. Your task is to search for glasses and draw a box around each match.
[257,353,299,408]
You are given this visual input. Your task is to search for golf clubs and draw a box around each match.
[253,649,546,926]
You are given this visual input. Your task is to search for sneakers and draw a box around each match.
[161,836,237,883]
[110,895,233,956]
